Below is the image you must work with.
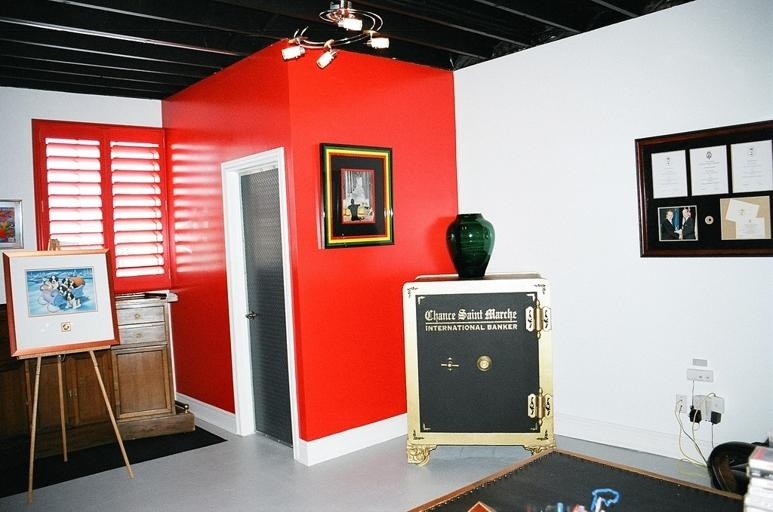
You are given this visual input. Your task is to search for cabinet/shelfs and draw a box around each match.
[0,292,196,469]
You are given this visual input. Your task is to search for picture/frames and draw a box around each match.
[318,140,395,250]
[633,120,772,259]
[1,199,25,249]
[2,250,121,360]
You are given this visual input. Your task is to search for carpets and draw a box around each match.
[2,426,228,501]
[409,447,744,512]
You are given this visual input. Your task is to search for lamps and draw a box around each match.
[279,0,391,70]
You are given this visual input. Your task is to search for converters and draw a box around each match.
[689,409,701,423]
[711,411,721,424]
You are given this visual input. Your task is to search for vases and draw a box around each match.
[445,213,496,278]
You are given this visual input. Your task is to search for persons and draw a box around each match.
[661,208,696,240]
